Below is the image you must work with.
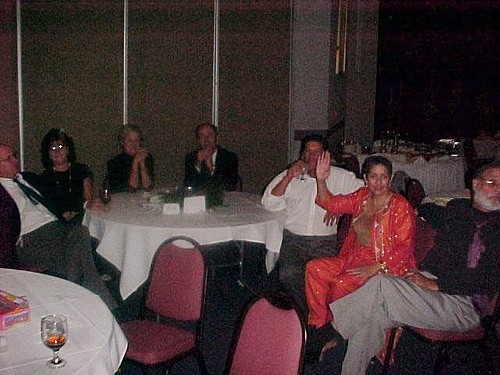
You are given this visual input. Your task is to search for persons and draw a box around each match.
[0,122,121,326]
[102,124,155,194]
[261,133,500,375]
[184,122,238,192]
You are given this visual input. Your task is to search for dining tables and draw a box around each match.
[81,187,284,299]
[421,189,470,214]
[345,153,466,196]
[0,267,128,375]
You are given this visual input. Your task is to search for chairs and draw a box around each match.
[117,236,208,375]
[380,282,500,374]
[222,290,308,375]
[405,179,426,209]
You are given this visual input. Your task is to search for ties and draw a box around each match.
[13,178,59,217]
[467,218,489,268]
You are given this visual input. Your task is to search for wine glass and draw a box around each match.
[41,314,69,369]
[100,188,112,211]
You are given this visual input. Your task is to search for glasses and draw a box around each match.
[48,144,66,150]
[480,177,500,188]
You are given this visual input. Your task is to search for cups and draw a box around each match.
[297,162,308,183]
